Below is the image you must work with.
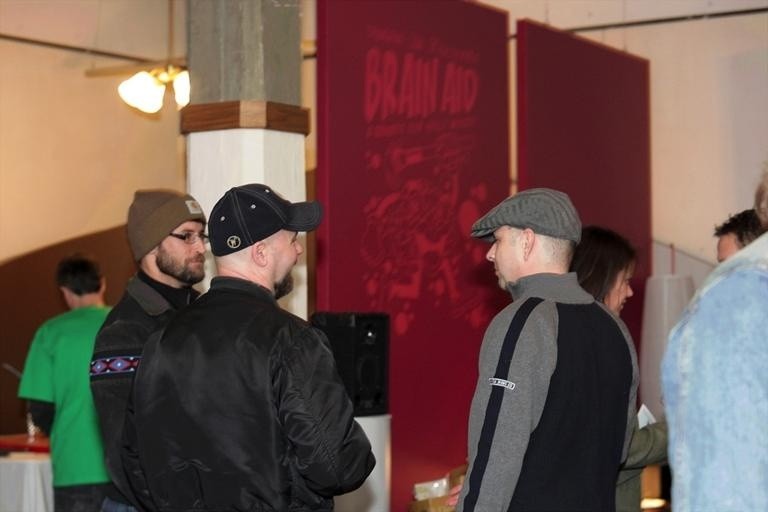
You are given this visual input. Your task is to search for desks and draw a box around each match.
[0,451,54,512]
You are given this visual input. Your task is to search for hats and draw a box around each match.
[128,189,205,264]
[209,183,324,256]
[470,188,583,244]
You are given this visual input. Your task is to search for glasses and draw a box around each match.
[167,231,208,245]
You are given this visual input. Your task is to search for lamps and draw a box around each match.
[116,61,192,115]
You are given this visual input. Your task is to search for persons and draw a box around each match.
[125,183,375,511]
[661,168,768,510]
[15,251,119,510]
[569,225,666,511]
[91,189,208,512]
[711,209,763,261]
[447,188,638,511]
